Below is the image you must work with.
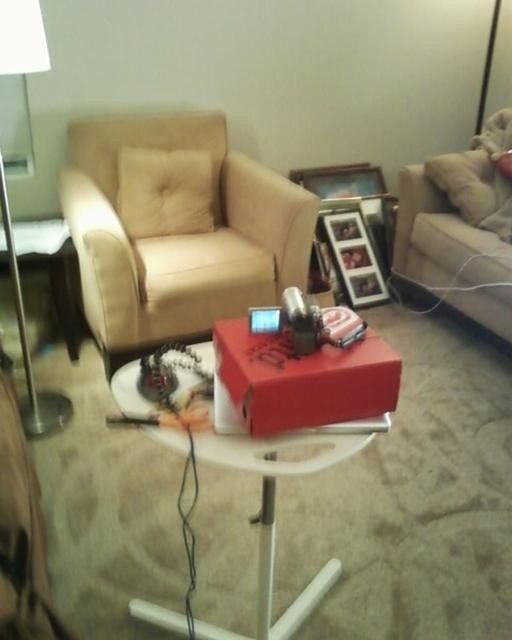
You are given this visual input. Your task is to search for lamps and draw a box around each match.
[0,0,74,441]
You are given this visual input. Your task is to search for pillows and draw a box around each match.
[420,146,506,227]
[107,137,220,238]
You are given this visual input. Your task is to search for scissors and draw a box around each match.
[105,405,213,429]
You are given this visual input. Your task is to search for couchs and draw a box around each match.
[386,104,512,353]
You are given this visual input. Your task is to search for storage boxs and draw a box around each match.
[211,303,404,440]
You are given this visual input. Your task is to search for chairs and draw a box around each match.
[53,104,325,378]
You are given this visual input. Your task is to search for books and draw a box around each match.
[213,373,392,434]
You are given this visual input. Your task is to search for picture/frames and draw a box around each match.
[288,160,394,314]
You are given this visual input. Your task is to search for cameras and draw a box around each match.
[248,286,322,356]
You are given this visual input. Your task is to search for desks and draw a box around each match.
[0,223,82,372]
